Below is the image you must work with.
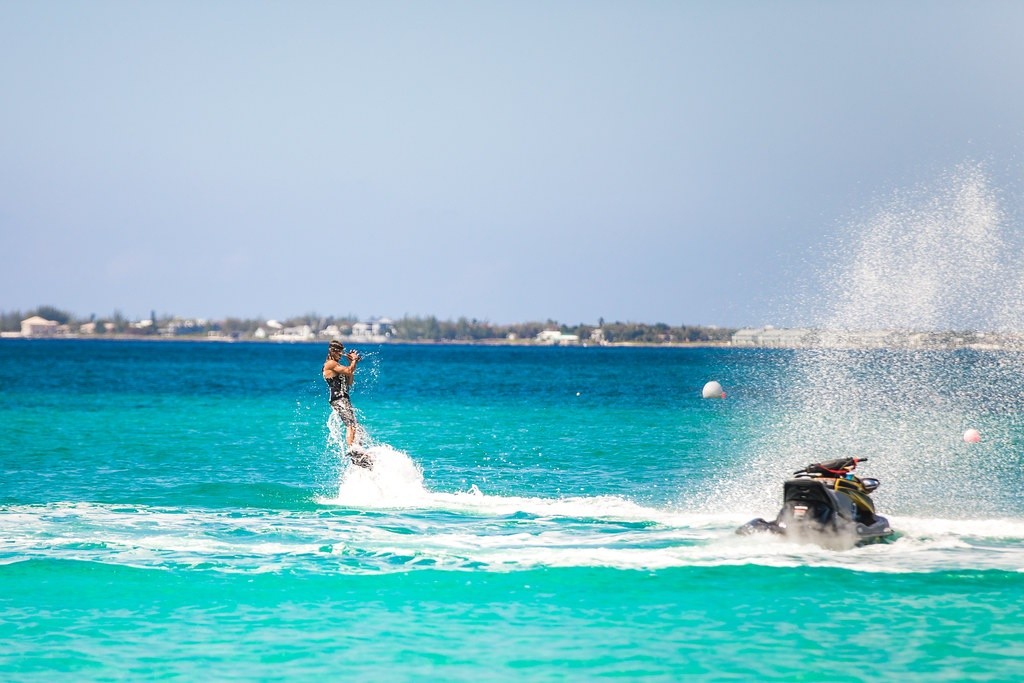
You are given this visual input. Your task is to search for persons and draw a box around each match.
[322,340,363,454]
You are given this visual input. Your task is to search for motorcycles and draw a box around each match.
[735,457,893,547]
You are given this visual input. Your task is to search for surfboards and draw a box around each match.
[347,451,374,470]
